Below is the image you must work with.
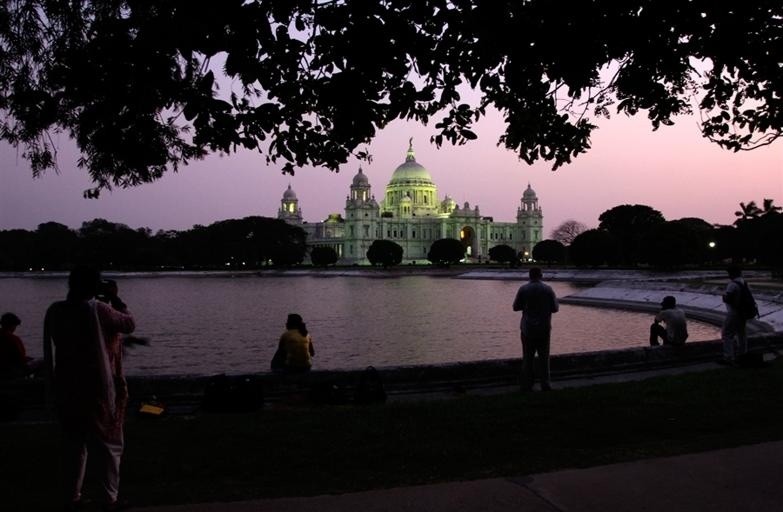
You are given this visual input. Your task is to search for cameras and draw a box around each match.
[97,281,109,295]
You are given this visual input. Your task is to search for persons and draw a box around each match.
[270,312,314,374]
[650,296,688,347]
[42,263,135,502]
[512,266,558,392]
[721,265,760,363]
[0,311,26,358]
